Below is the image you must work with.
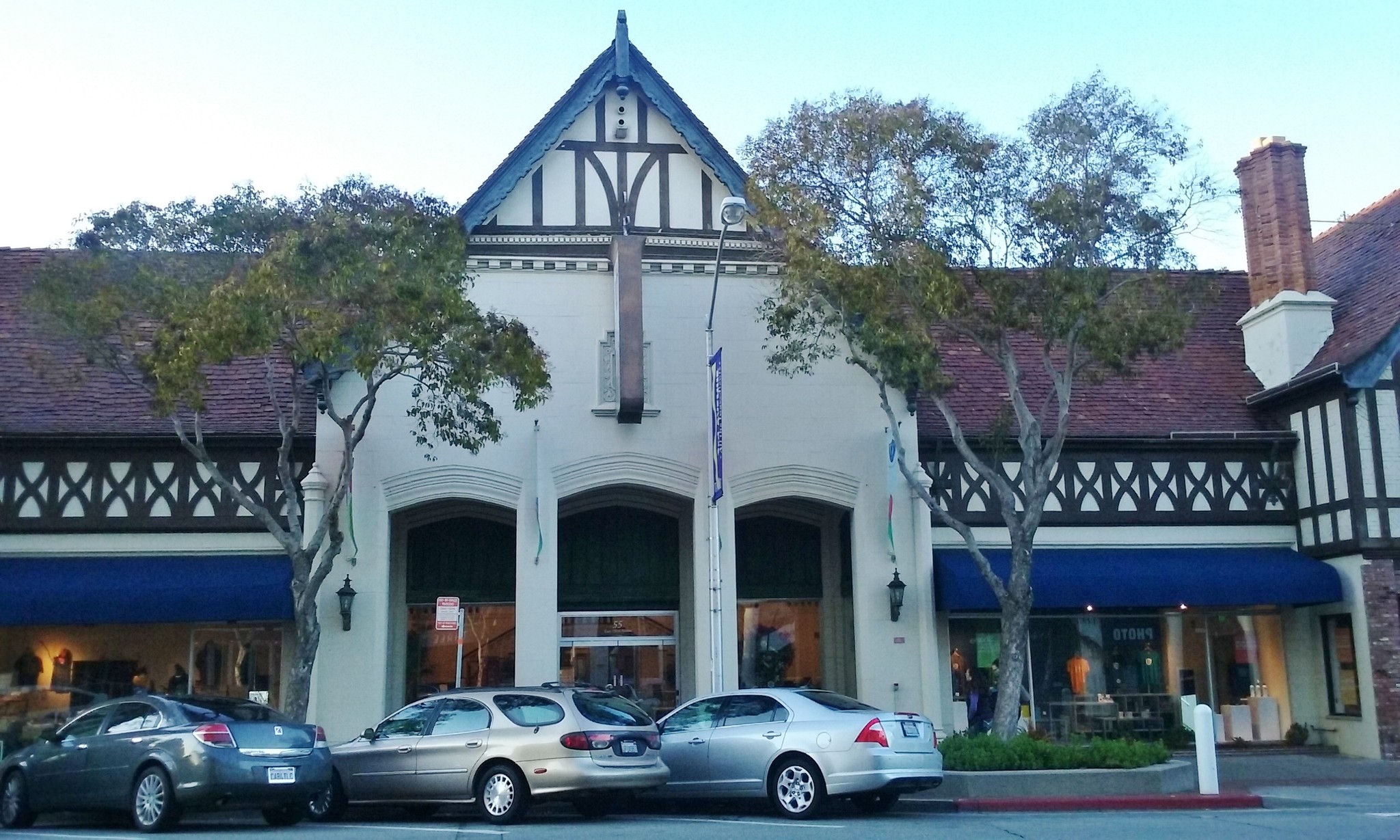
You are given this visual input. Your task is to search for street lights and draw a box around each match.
[705,193,747,696]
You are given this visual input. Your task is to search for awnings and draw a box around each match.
[931,545,1343,614]
[0,554,296,630]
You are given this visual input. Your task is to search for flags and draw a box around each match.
[708,347,724,501]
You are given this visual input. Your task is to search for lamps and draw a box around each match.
[335,574,358,630]
[885,568,907,622]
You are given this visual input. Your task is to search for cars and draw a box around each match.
[647,687,943,818]
[310,683,672,825]
[0,692,332,833]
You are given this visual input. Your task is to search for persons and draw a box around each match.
[169,664,196,696]
[965,665,994,738]
[992,659,1022,722]
